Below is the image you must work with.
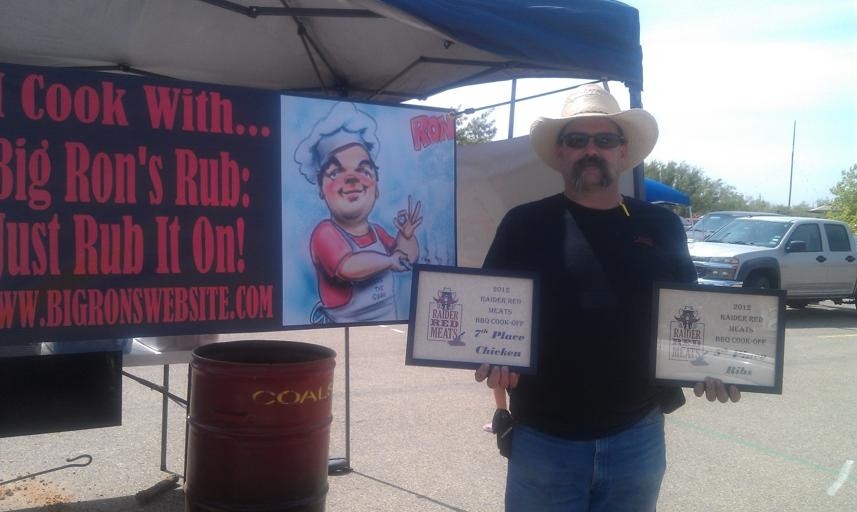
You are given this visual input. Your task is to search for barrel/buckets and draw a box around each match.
[184,340,336,512]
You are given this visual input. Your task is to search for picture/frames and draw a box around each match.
[648,280,787,394]
[405,263,541,374]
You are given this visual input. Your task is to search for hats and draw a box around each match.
[528,81,661,175]
[292,100,386,185]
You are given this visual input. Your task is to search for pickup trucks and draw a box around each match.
[686,211,857,308]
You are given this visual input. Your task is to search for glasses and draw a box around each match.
[561,131,623,151]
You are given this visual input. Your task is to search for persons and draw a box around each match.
[470,84,743,511]
[480,386,507,435]
